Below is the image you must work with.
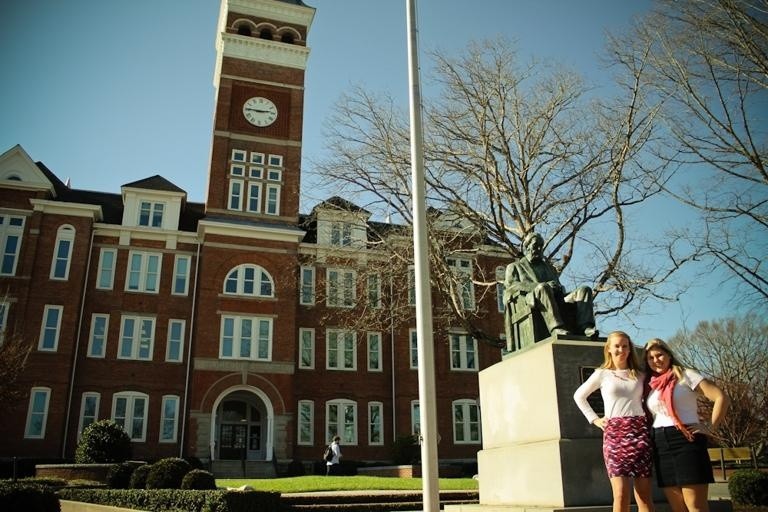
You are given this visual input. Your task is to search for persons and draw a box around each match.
[504,232,600,338]
[573,330,656,512]
[326,436,343,475]
[640,338,729,512]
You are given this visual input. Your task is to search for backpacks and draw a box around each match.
[323,443,334,462]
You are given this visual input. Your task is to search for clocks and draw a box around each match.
[242,96,278,127]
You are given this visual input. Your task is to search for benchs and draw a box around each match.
[707,446,758,480]
